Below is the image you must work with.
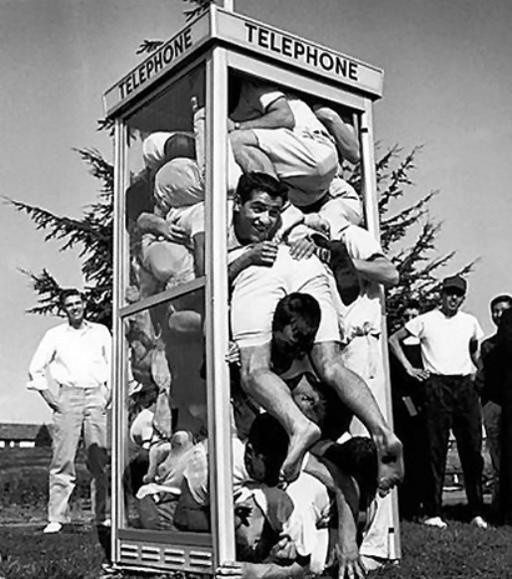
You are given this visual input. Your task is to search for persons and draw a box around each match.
[386,276,510,530]
[126,75,404,579]
[28,288,112,533]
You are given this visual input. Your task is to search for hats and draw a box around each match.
[443,276,466,291]
